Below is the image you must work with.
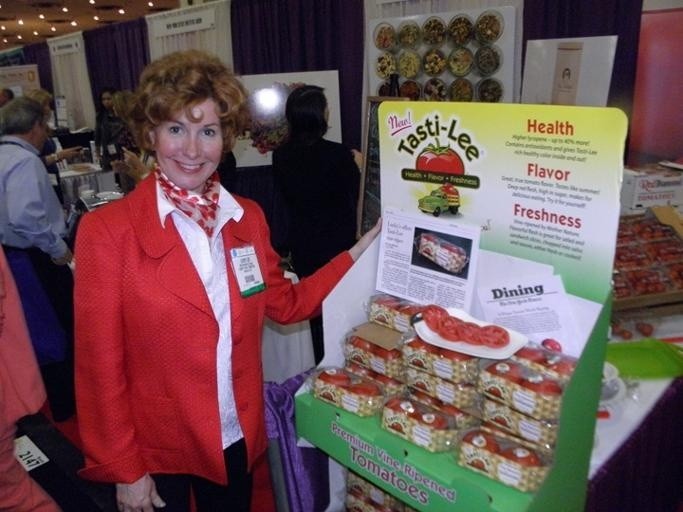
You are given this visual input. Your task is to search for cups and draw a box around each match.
[599,361,620,402]
[81,190,95,198]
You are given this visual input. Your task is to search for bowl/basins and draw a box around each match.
[372,8,506,102]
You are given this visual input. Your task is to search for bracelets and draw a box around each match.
[54,152,59,162]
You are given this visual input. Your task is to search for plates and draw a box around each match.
[599,378,627,407]
[96,191,122,200]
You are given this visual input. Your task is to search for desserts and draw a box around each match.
[319,293,577,481]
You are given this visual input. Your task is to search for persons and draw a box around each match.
[23,87,83,208]
[92,85,237,198]
[0,246,65,511]
[73,51,382,512]
[0,89,13,106]
[271,84,361,280]
[0,96,73,369]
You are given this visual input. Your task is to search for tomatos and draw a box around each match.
[612,216,683,340]
[414,136,465,174]
